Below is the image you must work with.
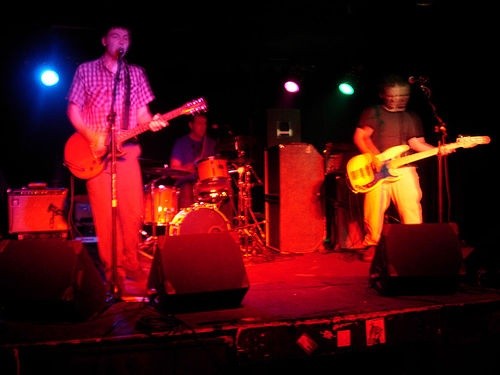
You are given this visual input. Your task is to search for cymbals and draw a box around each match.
[142,168,194,179]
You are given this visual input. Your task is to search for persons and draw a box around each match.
[172,114,216,211]
[67,23,169,300]
[354,78,456,262]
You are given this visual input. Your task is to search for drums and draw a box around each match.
[142,184,182,229]
[169,202,230,239]
[194,156,232,190]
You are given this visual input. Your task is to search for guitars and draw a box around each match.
[62,97,208,180]
[344,135,491,195]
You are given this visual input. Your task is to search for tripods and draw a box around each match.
[231,162,270,257]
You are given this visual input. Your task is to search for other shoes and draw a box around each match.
[361,245,376,261]
[106,267,149,281]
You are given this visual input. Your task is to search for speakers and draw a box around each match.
[0,189,111,326]
[262,106,328,255]
[145,232,249,310]
[367,223,463,296]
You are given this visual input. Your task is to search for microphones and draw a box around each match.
[408,76,427,84]
[118,47,124,55]
[48,204,63,215]
